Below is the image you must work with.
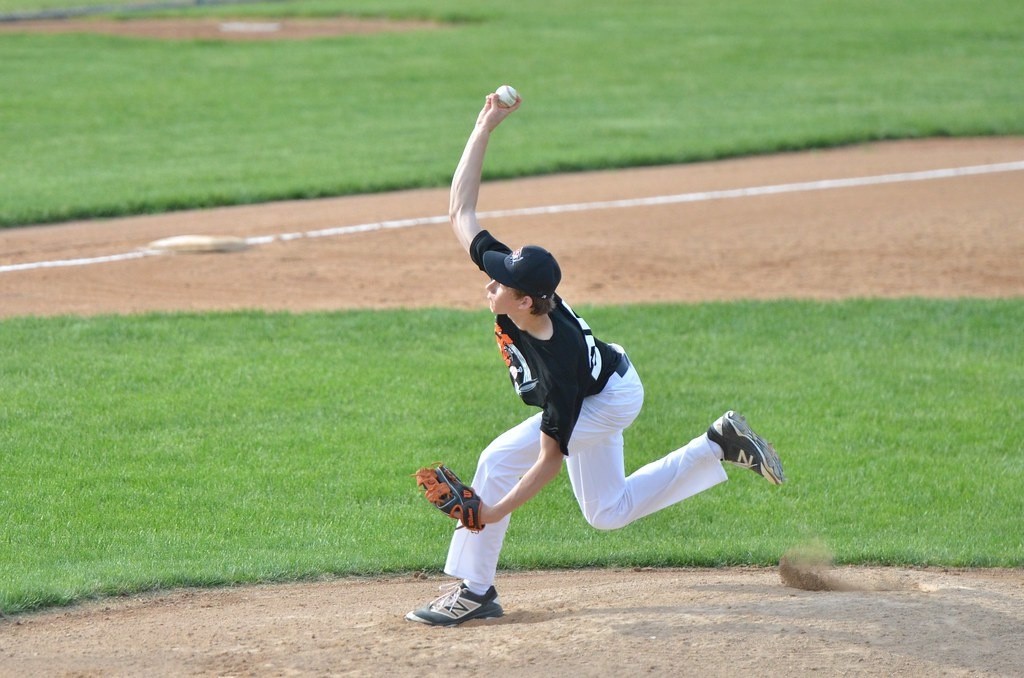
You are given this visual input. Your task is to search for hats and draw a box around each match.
[482,245,562,294]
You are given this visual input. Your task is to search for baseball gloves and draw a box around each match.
[415,463,486,532]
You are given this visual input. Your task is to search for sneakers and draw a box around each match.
[404,581,504,628]
[707,409,785,486]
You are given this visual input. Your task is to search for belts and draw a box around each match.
[616,353,630,377]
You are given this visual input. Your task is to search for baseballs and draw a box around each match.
[494,85,517,107]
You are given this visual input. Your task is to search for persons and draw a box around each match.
[405,93,786,625]
